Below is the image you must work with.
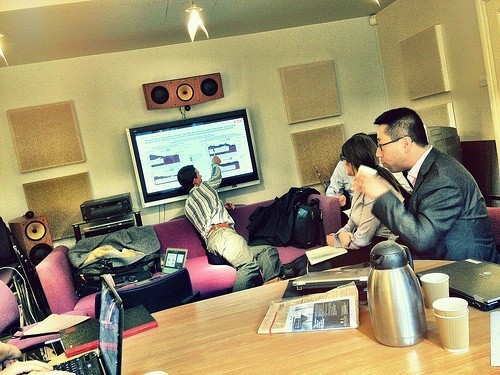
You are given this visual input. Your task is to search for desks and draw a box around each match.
[48,259,500,375]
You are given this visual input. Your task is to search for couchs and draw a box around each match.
[36,194,342,320]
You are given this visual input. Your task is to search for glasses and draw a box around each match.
[378,135,413,151]
[339,153,348,162]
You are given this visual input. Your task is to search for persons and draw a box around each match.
[374,107,500,264]
[326,133,410,265]
[178,155,284,293]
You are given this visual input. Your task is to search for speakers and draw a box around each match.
[142,73,225,110]
[8,215,54,272]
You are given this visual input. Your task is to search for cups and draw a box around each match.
[433,309,471,352]
[432,297,468,317]
[420,273,450,310]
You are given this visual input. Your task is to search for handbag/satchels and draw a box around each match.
[72,252,162,299]
[291,199,323,249]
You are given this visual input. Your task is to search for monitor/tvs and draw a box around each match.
[125,107,261,210]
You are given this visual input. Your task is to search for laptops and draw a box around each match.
[53,275,124,375]
[293,269,368,290]
[162,248,188,275]
[415,257,500,311]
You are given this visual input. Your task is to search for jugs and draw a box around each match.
[366,235,428,347]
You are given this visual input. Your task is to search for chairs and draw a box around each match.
[0,280,85,351]
[483,195,500,253]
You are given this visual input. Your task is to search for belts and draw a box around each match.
[206,223,233,236]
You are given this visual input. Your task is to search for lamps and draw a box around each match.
[186,2,208,43]
